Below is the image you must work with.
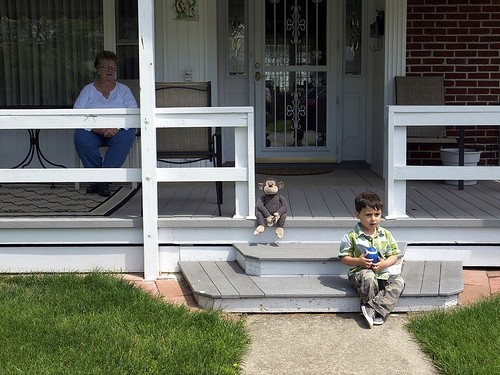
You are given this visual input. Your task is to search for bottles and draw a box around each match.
[366,247,378,263]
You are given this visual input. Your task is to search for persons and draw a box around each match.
[337,191,406,329]
[72,50,138,197]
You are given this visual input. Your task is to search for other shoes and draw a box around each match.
[95,183,110,197]
[86,184,97,193]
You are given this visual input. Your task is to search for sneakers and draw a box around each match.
[372,312,385,324]
[361,305,376,329]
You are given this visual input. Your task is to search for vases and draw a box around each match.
[440,148,481,186]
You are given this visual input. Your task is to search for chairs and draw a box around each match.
[395,76,464,190]
[75,80,140,189]
[155,80,223,204]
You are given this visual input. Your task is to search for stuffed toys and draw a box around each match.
[254,177,289,238]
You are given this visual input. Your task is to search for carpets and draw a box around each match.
[0,182,142,216]
[255,167,332,175]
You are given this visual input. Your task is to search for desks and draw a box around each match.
[0,105,73,169]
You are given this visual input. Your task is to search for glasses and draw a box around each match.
[98,64,117,72]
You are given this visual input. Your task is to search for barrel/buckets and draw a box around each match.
[441,148,483,185]
